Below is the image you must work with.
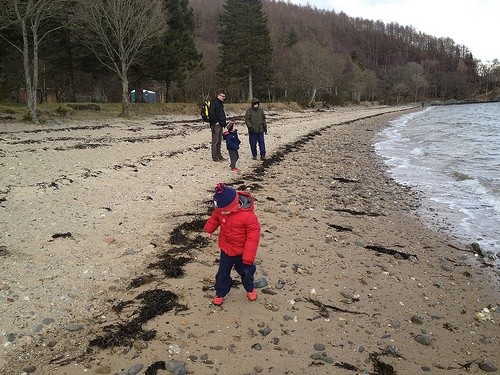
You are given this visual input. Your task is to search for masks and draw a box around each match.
[253,105,258,108]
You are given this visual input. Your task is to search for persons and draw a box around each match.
[208,89,227,162]
[244,98,269,161]
[203,183,261,306]
[222,121,241,172]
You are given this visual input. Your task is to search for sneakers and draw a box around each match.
[247,287,257,301]
[212,293,224,306]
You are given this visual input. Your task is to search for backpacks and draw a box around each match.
[201,98,218,122]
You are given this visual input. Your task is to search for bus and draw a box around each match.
[128,89,156,103]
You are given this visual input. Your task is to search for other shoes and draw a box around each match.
[261,155,266,160]
[212,157,225,161]
[228,164,240,172]
[253,156,257,160]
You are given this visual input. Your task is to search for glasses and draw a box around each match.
[219,94,226,98]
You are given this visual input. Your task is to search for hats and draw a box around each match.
[226,121,233,129]
[213,183,238,213]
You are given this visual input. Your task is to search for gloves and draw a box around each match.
[248,127,254,132]
[264,128,267,133]
